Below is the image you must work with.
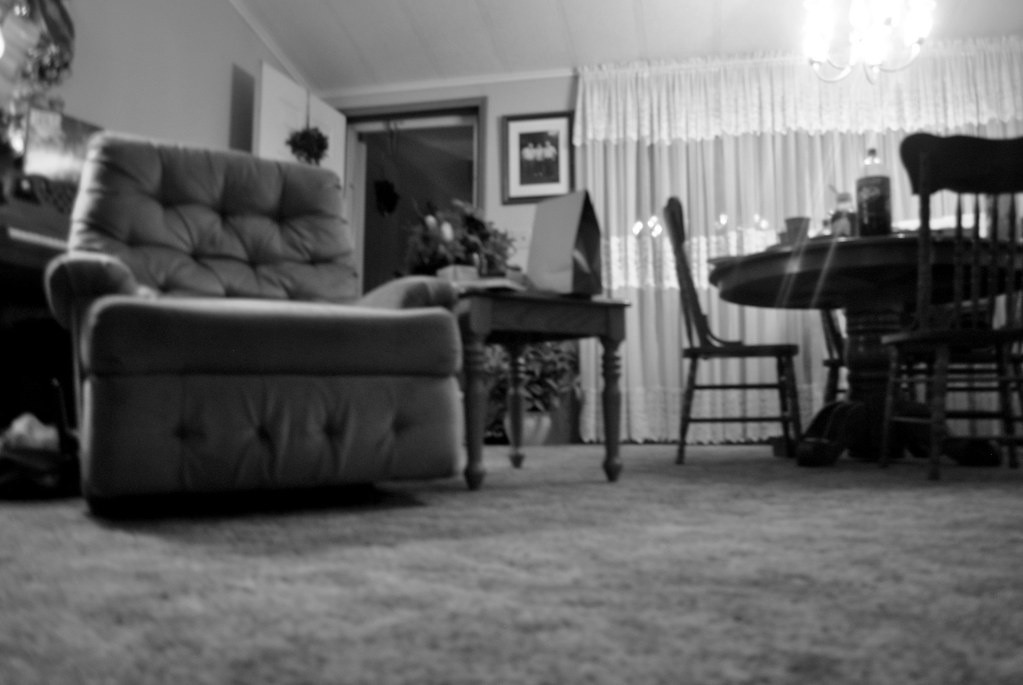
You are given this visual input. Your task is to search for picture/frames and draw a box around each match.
[501,108,575,205]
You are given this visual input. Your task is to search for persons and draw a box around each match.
[522,140,557,179]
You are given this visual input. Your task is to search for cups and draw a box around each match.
[786,217,809,242]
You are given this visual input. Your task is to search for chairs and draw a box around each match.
[816,306,851,405]
[876,130,1023,483]
[664,198,801,464]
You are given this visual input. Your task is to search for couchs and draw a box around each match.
[46,131,466,522]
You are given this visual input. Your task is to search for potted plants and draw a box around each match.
[402,197,515,284]
[487,334,579,445]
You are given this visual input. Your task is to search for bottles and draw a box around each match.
[830,192,856,236]
[855,149,892,237]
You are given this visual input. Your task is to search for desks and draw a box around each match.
[446,290,631,494]
[707,232,1023,467]
